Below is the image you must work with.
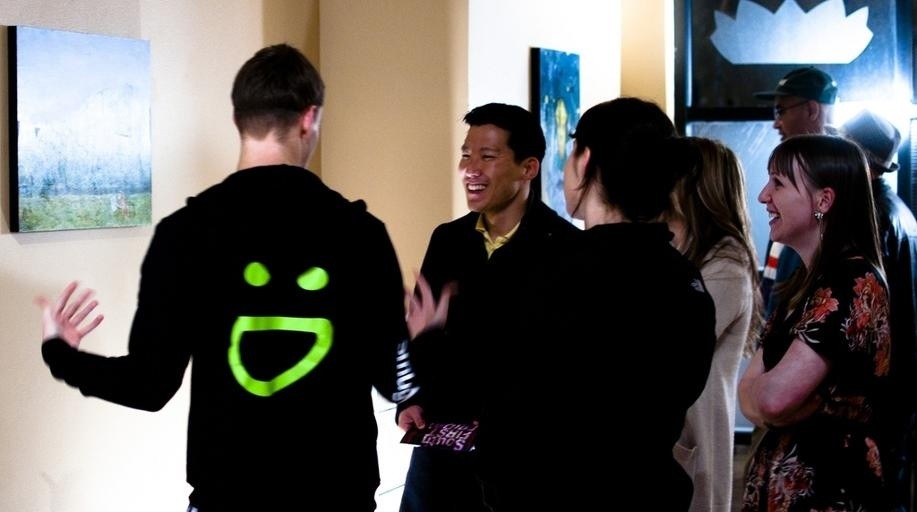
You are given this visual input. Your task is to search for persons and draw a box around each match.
[403,98,715,512]
[755,66,839,303]
[845,112,917,440]
[738,136,889,512]
[34,43,416,512]
[396,104,585,512]
[673,137,760,511]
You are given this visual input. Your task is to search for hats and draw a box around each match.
[824,112,901,176]
[754,67,837,104]
